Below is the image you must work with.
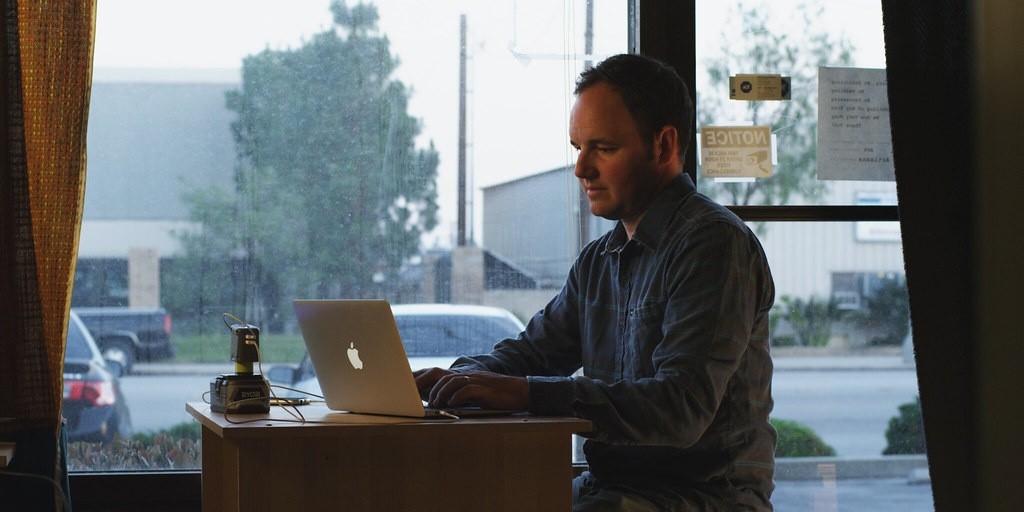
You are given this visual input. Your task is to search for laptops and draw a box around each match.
[293,298,526,419]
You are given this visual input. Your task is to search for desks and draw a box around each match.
[185,400,593,511]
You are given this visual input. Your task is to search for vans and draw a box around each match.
[63,307,174,367]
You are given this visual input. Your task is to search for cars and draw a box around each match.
[62,308,130,449]
[269,303,524,399]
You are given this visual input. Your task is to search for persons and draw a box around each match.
[413,54,778,512]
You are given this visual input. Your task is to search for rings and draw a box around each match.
[464,376,469,384]
